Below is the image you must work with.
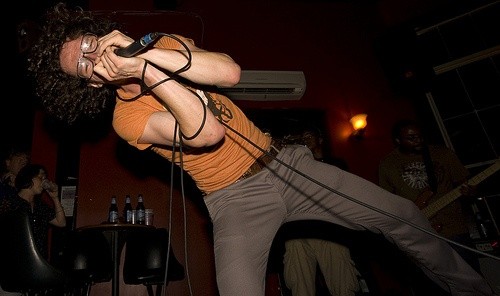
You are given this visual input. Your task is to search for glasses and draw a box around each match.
[77,33,98,80]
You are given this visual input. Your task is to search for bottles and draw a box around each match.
[136,193,145,225]
[108,196,119,224]
[123,194,132,224]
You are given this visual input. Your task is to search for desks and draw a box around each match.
[77,224,156,296]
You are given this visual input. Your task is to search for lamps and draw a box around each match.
[349,114,368,138]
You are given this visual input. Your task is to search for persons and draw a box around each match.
[379,120,486,296]
[0,145,66,296]
[22,4,493,296]
[282,130,358,296]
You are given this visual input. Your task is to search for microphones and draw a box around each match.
[114,33,157,58]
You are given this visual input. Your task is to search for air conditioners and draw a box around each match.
[218,70,306,101]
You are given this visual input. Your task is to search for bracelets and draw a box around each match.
[141,60,147,87]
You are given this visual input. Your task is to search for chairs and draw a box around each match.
[0,198,125,296]
[123,227,185,296]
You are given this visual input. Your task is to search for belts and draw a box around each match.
[234,139,282,185]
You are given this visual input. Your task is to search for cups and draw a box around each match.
[144,209,153,225]
[42,179,58,192]
[131,210,136,225]
